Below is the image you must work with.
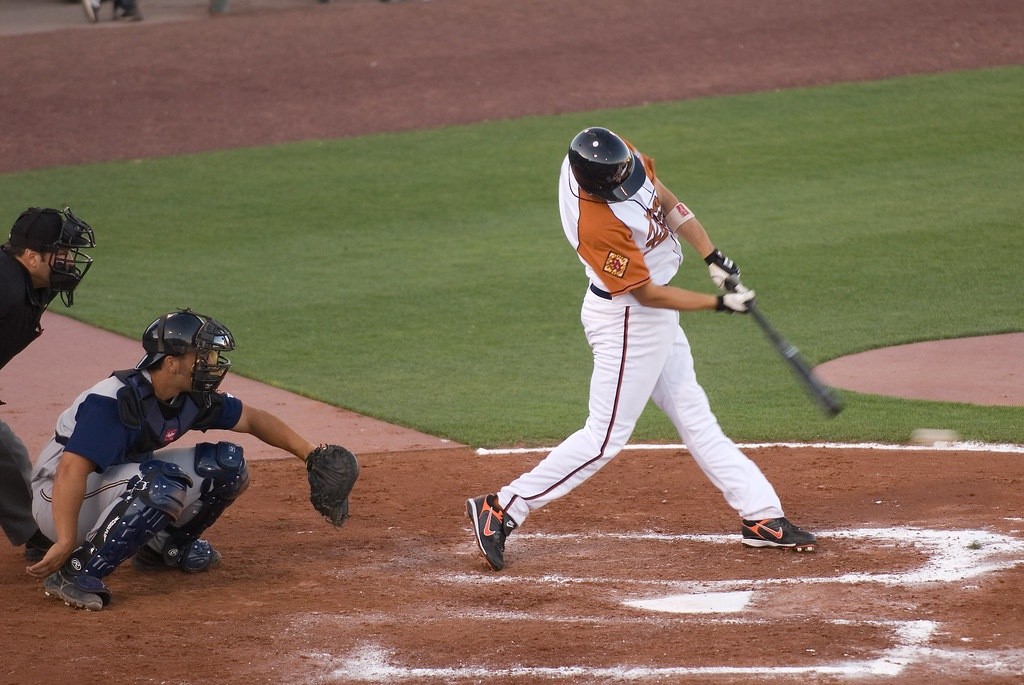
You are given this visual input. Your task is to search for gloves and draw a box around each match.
[715,290,756,316]
[704,248,741,291]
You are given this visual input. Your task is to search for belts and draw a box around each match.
[590,283,612,300]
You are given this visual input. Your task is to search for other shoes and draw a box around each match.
[25,535,83,560]
[113,0,143,23]
[84,0,97,23]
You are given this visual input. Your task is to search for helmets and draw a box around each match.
[135,308,235,370]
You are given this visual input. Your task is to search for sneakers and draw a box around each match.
[43,570,103,611]
[134,543,222,570]
[741,517,816,552]
[466,493,518,572]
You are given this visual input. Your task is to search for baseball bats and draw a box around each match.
[724,274,847,418]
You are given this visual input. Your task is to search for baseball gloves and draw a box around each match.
[304,443,360,527]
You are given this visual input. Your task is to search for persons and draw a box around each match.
[25,307,360,612]
[466,128,819,572]
[0,207,96,562]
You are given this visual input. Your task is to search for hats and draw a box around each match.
[9,207,89,252]
[569,127,646,202]
